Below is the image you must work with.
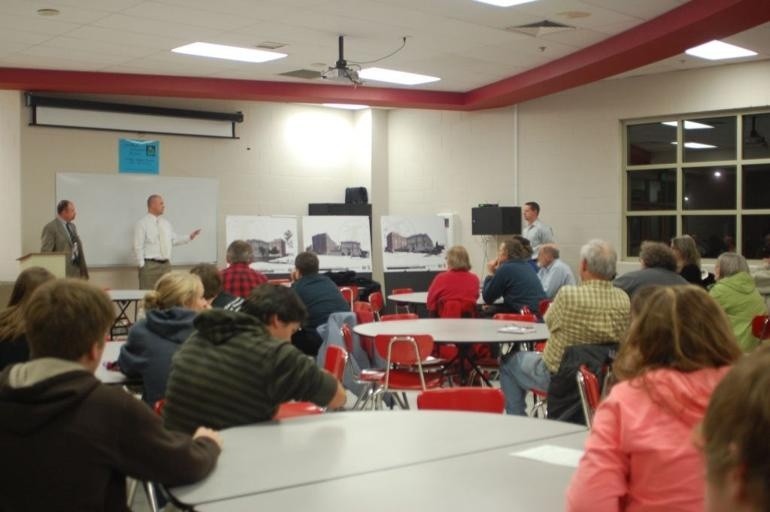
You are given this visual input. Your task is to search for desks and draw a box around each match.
[163,409,589,511]
[92,340,128,387]
[101,289,157,342]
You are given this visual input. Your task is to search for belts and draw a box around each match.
[144,259,168,263]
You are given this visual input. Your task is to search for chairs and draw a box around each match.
[126,396,171,511]
[748,314,769,345]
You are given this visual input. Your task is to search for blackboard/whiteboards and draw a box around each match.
[55,171,218,267]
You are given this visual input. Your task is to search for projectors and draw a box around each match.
[318,68,358,84]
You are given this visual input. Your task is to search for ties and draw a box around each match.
[66,222,80,264]
[157,217,165,257]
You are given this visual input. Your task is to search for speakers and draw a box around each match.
[471,206,522,236]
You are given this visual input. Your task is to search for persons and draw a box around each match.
[2,269,58,368]
[500,239,632,416]
[0,276,223,511]
[159,283,348,435]
[118,238,349,410]
[704,339,769,511]
[670,235,703,287]
[135,194,200,289]
[562,284,750,512]
[425,245,480,318]
[41,199,88,282]
[521,202,555,263]
[482,236,577,320]
[708,249,768,354]
[613,242,690,299]
